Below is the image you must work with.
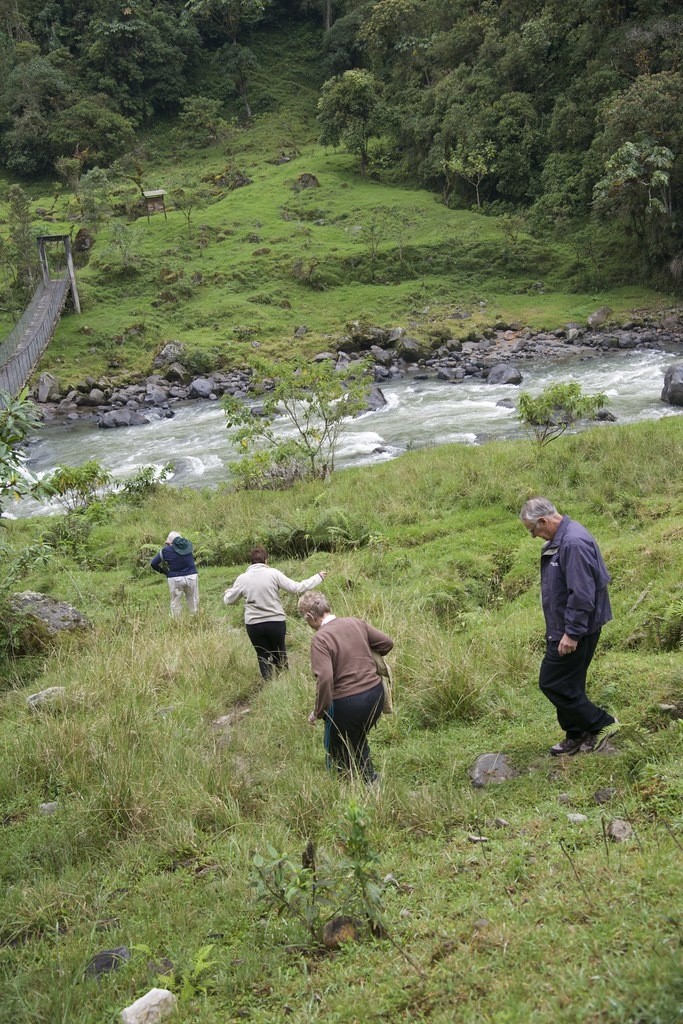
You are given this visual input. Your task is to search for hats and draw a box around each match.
[172,536,192,554]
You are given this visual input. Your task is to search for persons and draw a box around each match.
[297,590,394,785]
[520,497,622,756]
[150,532,198,623]
[223,548,326,682]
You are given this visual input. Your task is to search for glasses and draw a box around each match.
[529,522,539,537]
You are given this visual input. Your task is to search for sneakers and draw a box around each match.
[580,718,620,755]
[551,730,589,756]
